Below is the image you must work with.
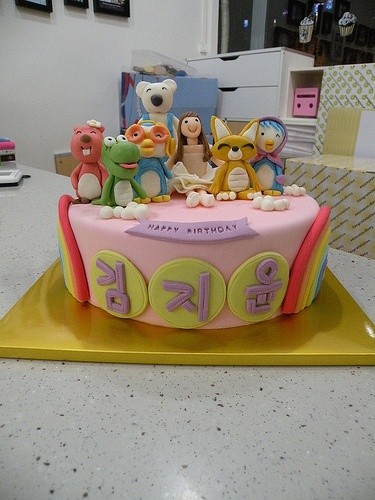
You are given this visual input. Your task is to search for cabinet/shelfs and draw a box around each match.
[185,47,316,121]
[282,66,324,156]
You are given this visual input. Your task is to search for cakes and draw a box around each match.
[58,79,331,330]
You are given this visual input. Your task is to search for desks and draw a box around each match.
[0,159,375,500]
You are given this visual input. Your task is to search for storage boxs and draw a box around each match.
[294,86,320,117]
[284,106,375,260]
[119,71,218,143]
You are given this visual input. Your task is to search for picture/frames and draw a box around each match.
[93,0,130,18]
[64,0,89,9]
[14,0,53,13]
